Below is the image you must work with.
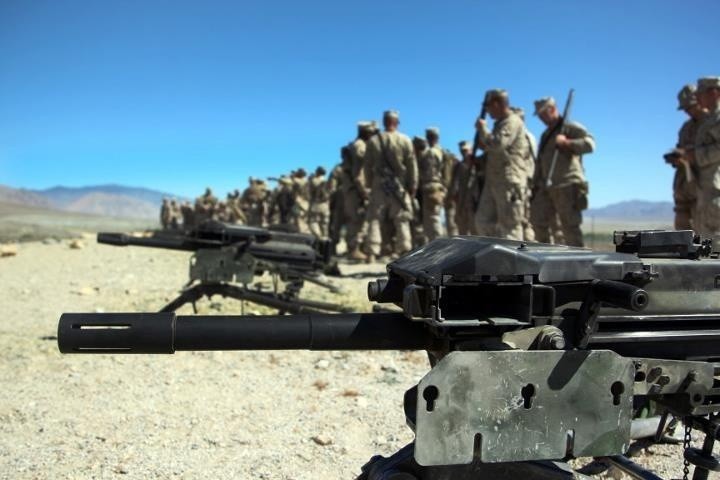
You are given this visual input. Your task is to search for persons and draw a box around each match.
[671,84,709,229]
[683,77,720,252]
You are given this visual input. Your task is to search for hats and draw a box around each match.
[678,77,720,109]
[357,110,398,134]
[297,167,326,175]
[459,140,473,149]
[413,127,439,145]
[484,88,523,116]
[533,98,554,113]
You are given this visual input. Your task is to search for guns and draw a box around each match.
[57,230,719,368]
[546,89,574,186]
[464,95,487,193]
[98,220,333,282]
